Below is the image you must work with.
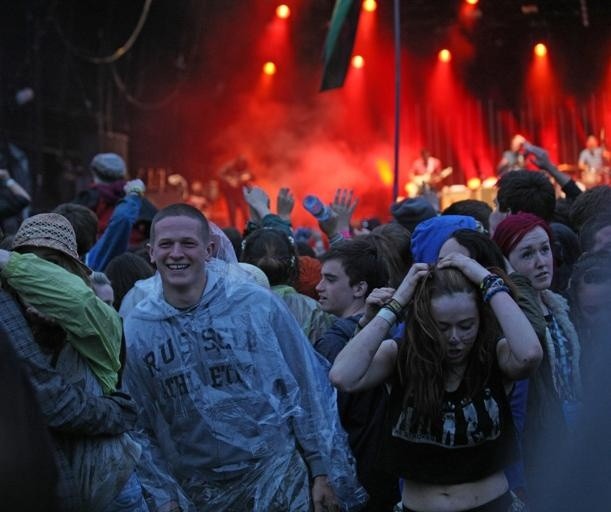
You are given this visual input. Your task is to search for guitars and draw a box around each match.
[405,166,451,198]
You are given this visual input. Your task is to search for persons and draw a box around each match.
[0,134,611,512]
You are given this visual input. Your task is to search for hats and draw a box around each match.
[390,197,438,233]
[10,212,92,278]
[91,153,126,183]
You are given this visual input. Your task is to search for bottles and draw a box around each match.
[301,194,330,222]
